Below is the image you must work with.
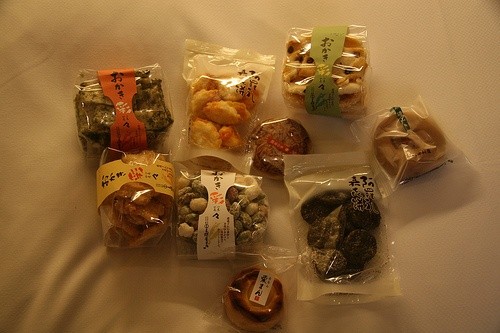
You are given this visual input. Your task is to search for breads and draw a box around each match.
[189,72,259,150]
[222,269,283,333]
[374,111,446,181]
[299,189,379,282]
